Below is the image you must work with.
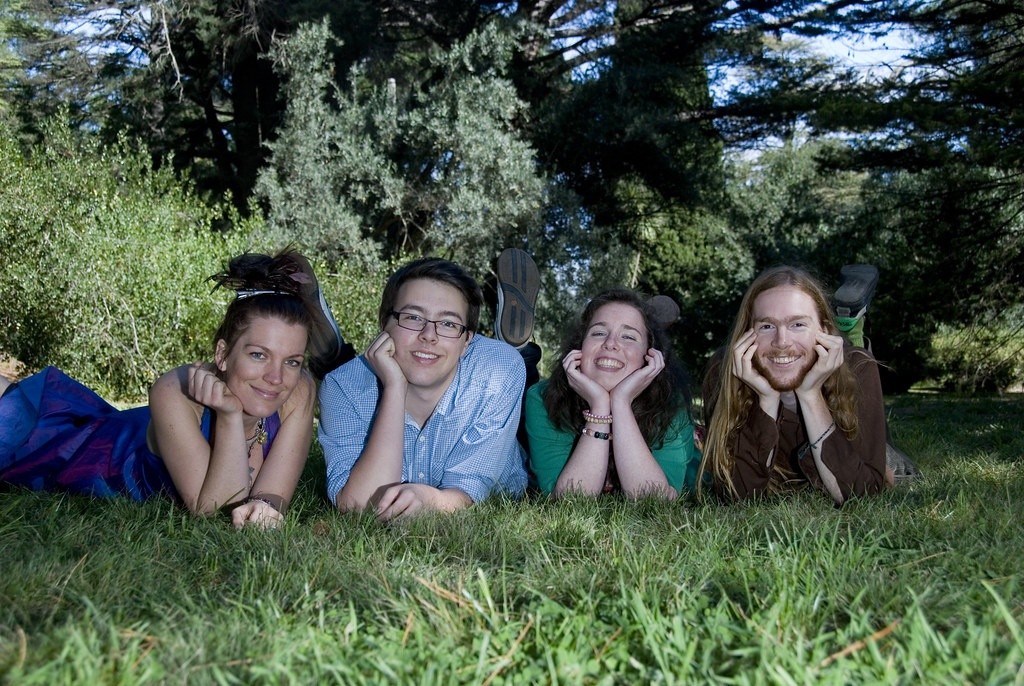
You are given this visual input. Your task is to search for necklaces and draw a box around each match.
[245,414,269,458]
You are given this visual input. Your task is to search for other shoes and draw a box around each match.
[491,248,541,349]
[832,265,879,332]
[275,252,343,366]
[644,295,680,331]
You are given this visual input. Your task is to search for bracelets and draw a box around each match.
[800,421,835,459]
[251,497,279,510]
[582,409,612,424]
[582,428,612,440]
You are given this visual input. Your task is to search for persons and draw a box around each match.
[295,247,543,522]
[523,287,705,504]
[694,260,915,510]
[0,251,317,533]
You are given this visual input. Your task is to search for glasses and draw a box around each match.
[391,310,468,338]
